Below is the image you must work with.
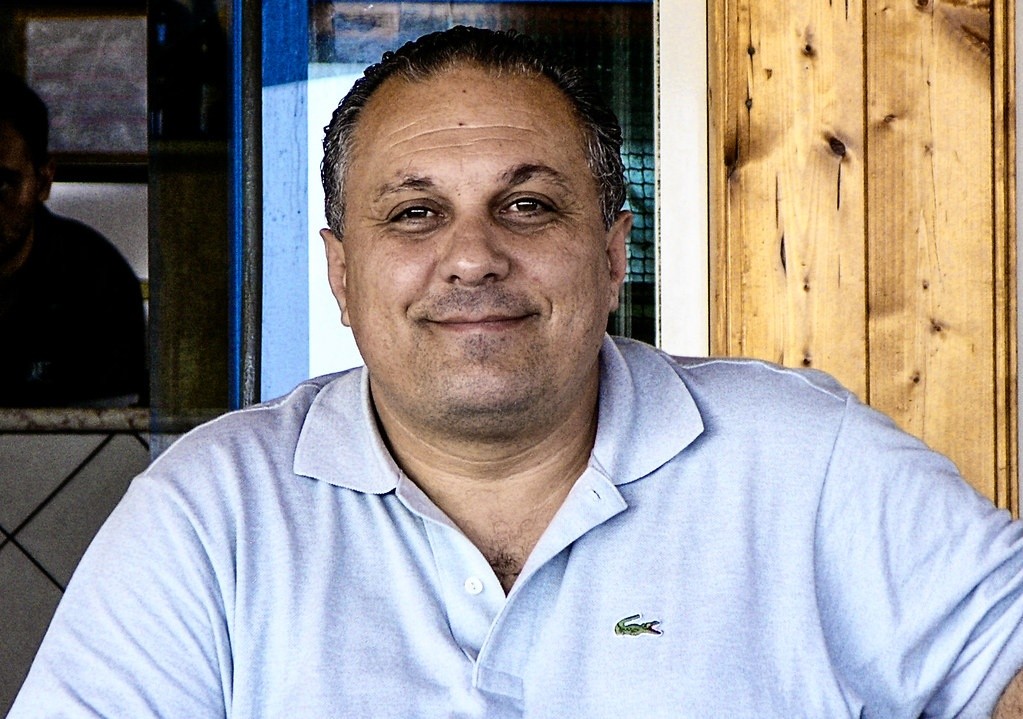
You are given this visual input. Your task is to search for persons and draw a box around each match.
[3,25,1023,719]
[0,73,149,411]
